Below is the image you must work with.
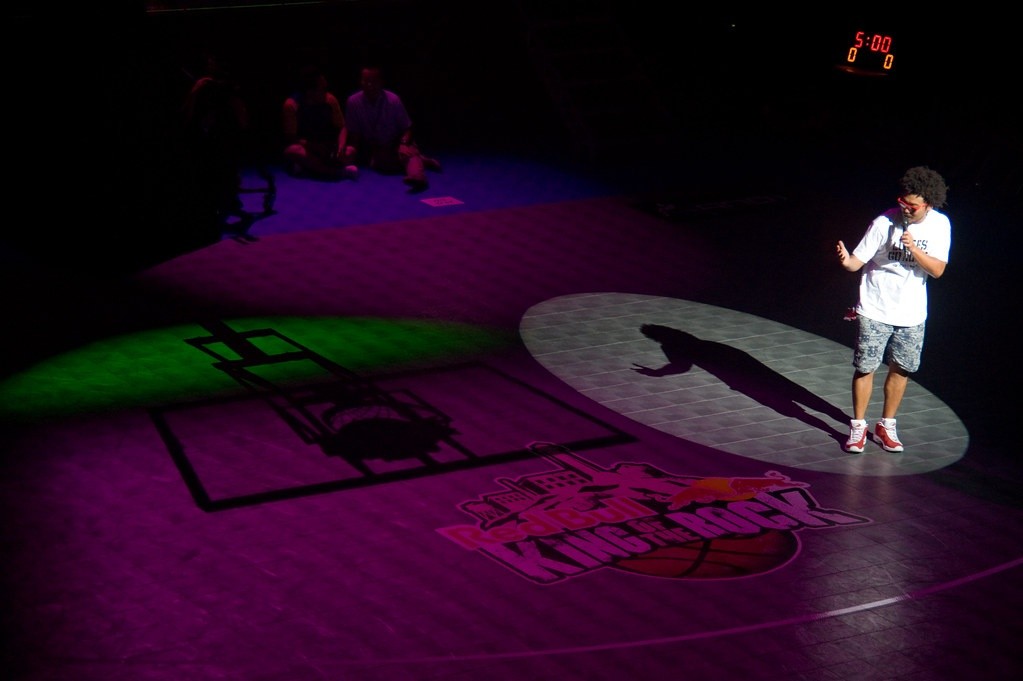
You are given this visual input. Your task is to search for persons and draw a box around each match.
[837,166,951,452]
[282,70,360,177]
[344,65,412,167]
[177,52,230,124]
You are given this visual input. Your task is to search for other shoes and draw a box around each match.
[291,162,303,175]
[403,174,428,186]
[337,165,359,178]
[420,154,441,167]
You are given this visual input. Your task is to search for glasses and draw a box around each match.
[898,197,929,213]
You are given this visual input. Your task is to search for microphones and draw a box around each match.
[902,217,909,259]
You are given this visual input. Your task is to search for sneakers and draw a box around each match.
[846,419,869,452]
[873,418,904,451]
